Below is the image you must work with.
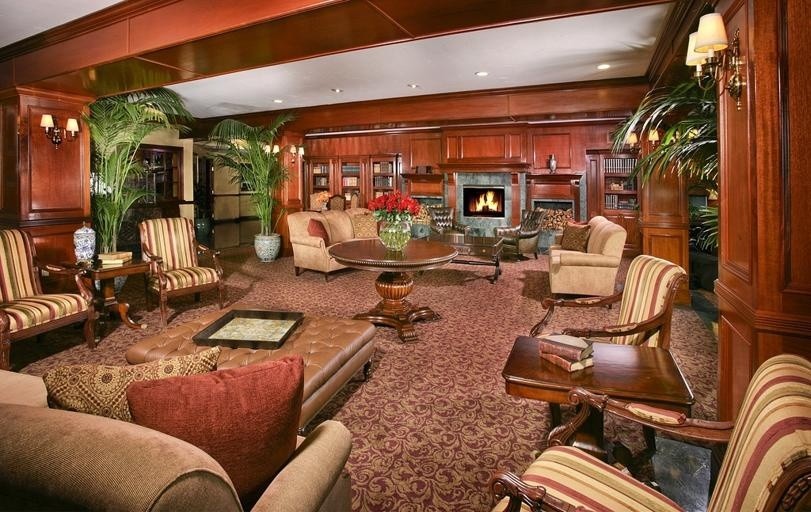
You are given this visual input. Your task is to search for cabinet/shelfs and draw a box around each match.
[367,152,403,201]
[303,153,335,209]
[598,151,641,258]
[335,153,367,209]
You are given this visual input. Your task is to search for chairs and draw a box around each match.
[326,194,346,210]
[483,353,810,511]
[524,252,691,458]
[349,192,360,208]
[136,216,225,329]
[0,226,99,371]
[491,206,545,265]
[428,205,474,243]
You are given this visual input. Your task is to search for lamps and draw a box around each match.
[287,144,306,164]
[683,11,744,113]
[38,113,82,151]
[626,128,661,152]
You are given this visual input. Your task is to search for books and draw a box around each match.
[310,163,394,200]
[604,157,637,209]
[536,332,596,361]
[97,251,132,268]
[540,350,595,373]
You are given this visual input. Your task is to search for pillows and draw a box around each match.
[348,212,379,239]
[124,352,307,512]
[307,218,332,248]
[561,220,591,253]
[560,216,591,246]
[41,343,223,424]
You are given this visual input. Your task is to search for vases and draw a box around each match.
[547,153,558,174]
[376,220,412,251]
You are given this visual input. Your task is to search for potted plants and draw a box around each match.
[200,109,308,264]
[77,86,196,295]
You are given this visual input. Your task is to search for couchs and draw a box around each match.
[285,206,379,284]
[0,343,356,512]
[546,213,629,312]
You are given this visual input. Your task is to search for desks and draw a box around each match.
[327,236,459,347]
[38,254,153,332]
[500,334,696,467]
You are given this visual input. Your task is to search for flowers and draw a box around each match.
[366,189,422,250]
